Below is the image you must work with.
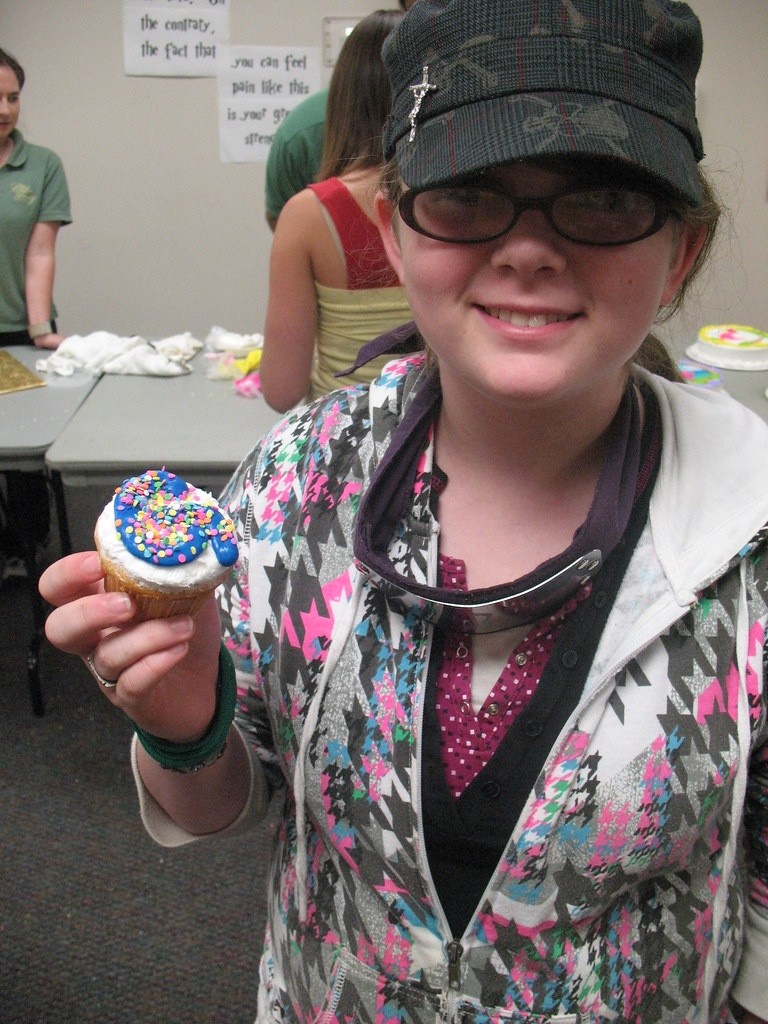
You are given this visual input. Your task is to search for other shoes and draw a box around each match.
[2,556,28,581]
[33,537,54,564]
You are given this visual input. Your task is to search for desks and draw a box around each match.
[0,342,768,719]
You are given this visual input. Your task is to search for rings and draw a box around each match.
[87,641,118,687]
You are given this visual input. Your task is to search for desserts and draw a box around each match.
[94,465,240,628]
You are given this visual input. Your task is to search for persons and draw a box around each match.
[36,0,768,1024]
[260,10,428,415]
[0,47,72,348]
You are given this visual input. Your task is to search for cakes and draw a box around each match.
[695,323,768,368]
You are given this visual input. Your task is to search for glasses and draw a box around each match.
[393,181,674,246]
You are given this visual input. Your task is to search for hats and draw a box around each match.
[381,0,704,207]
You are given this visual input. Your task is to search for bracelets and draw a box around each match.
[28,321,52,339]
[129,641,237,772]
